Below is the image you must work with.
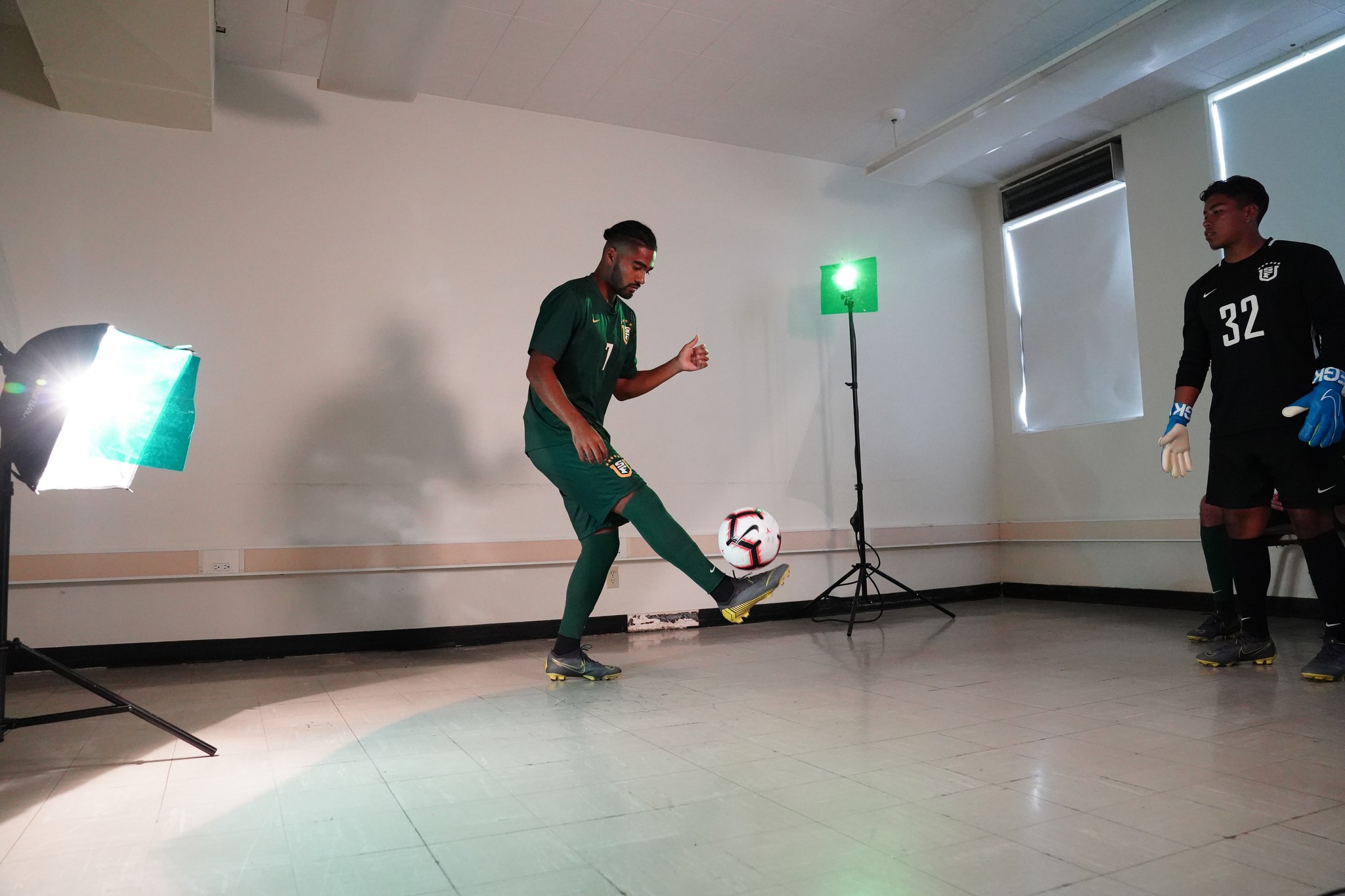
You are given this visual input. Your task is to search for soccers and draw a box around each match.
[718,506,781,571]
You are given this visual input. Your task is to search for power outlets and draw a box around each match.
[198,550,239,574]
[607,566,618,589]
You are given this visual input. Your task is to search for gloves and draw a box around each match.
[1281,367,1345,448]
[1157,402,1194,479]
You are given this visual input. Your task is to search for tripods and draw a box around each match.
[800,304,958,637]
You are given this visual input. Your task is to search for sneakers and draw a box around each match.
[1193,632,1277,668]
[545,644,622,682]
[1185,613,1242,643]
[1300,633,1345,682]
[714,563,791,625]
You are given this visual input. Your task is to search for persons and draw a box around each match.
[1185,492,1283,643]
[1162,176,1345,685]
[523,221,788,682]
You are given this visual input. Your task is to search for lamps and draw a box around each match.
[865,0,1301,188]
[317,0,449,103]
